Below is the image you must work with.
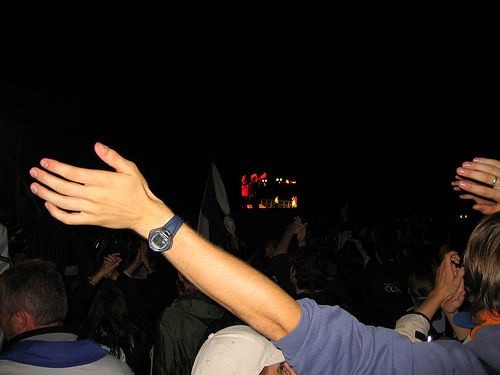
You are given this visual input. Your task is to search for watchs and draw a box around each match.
[148,214,185,253]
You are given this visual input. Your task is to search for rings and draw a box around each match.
[491,176,498,185]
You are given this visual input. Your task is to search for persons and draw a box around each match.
[452,150,500,217]
[0,194,473,375]
[28,142,500,375]
